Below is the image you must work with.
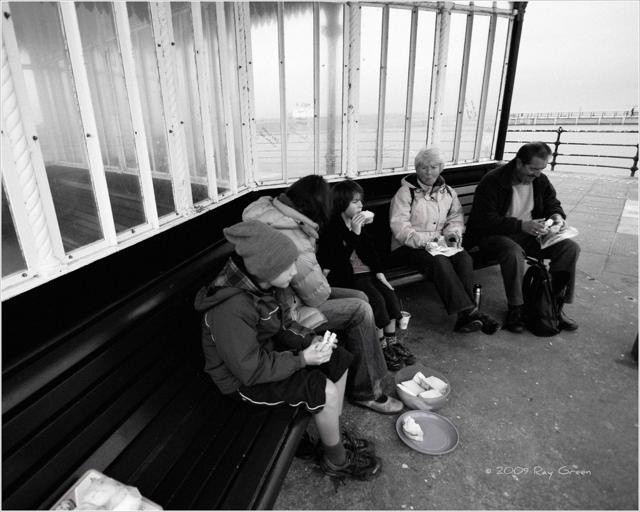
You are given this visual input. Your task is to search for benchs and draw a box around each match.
[2,180,499,510]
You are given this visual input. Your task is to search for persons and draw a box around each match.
[195,219,383,480]
[242,175,404,415]
[389,147,501,338]
[472,141,582,337]
[310,180,415,371]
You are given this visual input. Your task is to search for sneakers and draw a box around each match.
[319,449,382,479]
[315,431,371,457]
[381,344,417,373]
[453,313,503,336]
[504,306,526,336]
[557,309,579,333]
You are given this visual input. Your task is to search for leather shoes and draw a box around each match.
[346,394,405,415]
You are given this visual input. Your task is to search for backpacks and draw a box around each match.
[521,256,562,337]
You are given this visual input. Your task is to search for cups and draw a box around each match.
[397,310,412,331]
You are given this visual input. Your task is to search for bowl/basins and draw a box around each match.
[395,365,451,411]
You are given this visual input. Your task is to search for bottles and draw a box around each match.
[473,284,481,309]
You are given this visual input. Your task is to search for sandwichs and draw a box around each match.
[316,330,337,354]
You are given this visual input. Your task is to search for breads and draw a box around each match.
[403,414,424,444]
[398,372,448,413]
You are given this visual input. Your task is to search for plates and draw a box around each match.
[395,409,460,457]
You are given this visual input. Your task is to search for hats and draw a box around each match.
[221,220,300,284]
